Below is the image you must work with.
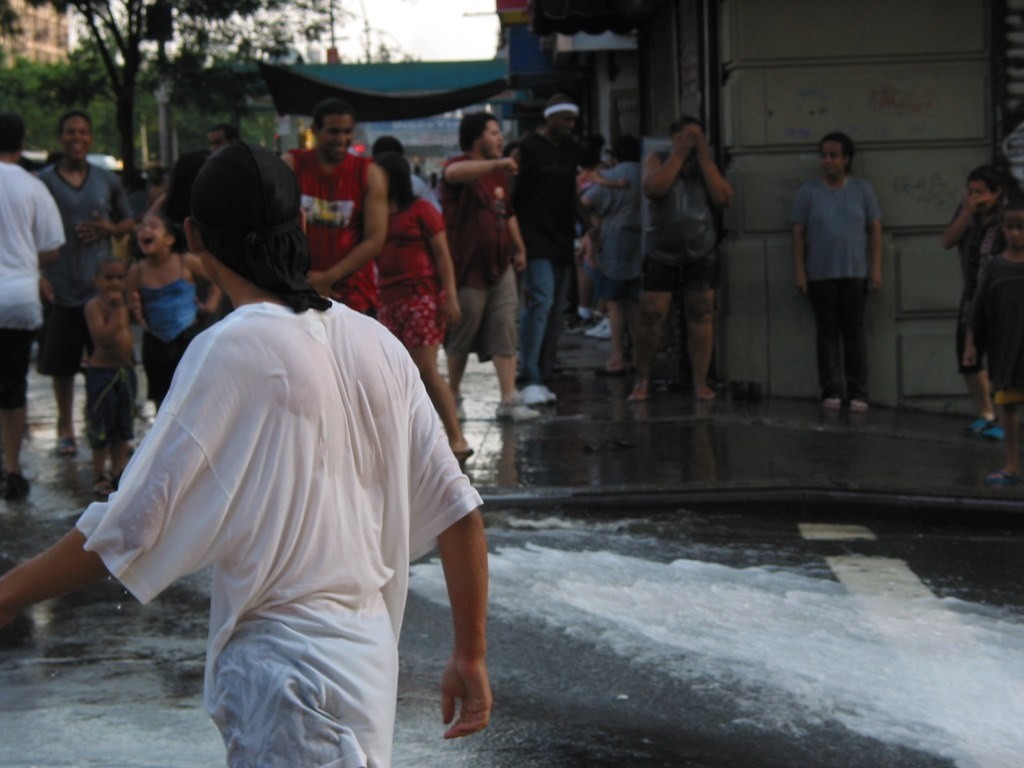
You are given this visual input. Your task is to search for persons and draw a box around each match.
[0,93,734,500]
[942,164,1024,483]
[0,140,493,768]
[792,132,884,412]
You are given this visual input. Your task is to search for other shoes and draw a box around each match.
[590,365,627,377]
[628,380,651,402]
[584,316,611,340]
[0,472,30,499]
[694,385,717,401]
[563,317,593,334]
[848,397,869,413]
[496,403,541,423]
[57,437,76,457]
[517,385,557,407]
[92,469,124,501]
[454,397,467,422]
[823,397,842,411]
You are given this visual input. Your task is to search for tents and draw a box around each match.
[152,56,517,139]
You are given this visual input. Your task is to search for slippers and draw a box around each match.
[449,440,473,458]
[983,427,1005,440]
[965,416,999,433]
[985,469,1021,484]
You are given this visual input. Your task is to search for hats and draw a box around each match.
[542,91,579,120]
